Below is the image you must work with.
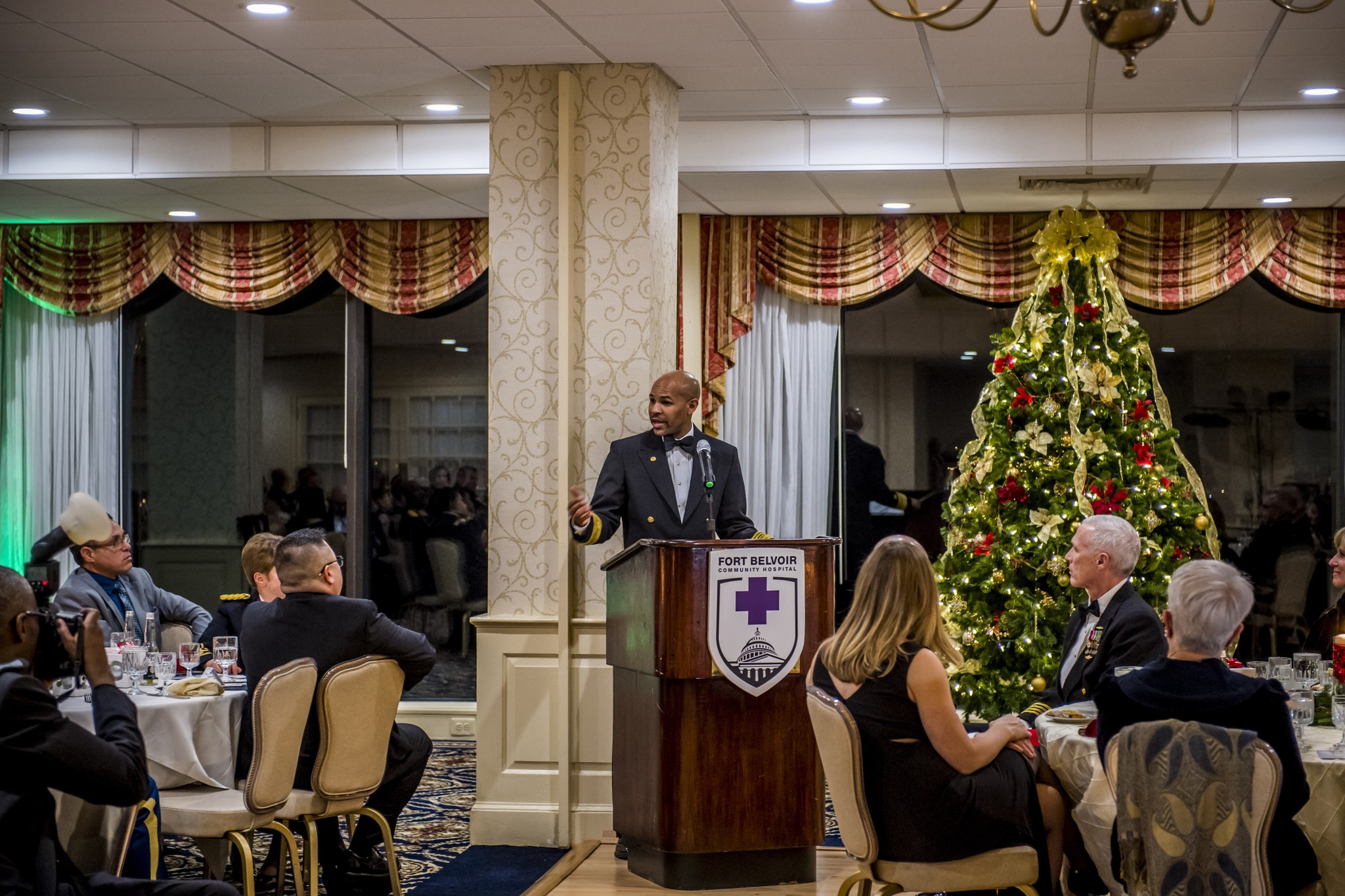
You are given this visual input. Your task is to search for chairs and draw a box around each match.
[144,658,308,896]
[1103,717,1324,896]
[805,686,1043,896]
[273,653,411,896]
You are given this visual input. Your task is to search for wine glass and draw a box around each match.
[1294,652,1321,690]
[111,631,133,648]
[1331,695,1345,748]
[179,642,201,678]
[1317,661,1333,693]
[1287,689,1313,749]
[213,636,238,682]
[1268,656,1292,685]
[154,653,176,696]
[122,646,148,696]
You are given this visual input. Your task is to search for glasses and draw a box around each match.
[9,607,50,626]
[85,533,130,551]
[318,556,344,577]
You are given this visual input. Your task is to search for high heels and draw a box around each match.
[1067,867,1111,896]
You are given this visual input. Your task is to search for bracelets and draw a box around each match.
[967,731,977,739]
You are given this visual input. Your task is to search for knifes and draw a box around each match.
[146,693,190,699]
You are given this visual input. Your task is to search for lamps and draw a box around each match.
[871,0,1334,83]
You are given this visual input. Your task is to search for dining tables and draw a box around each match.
[1032,700,1345,896]
[51,666,248,872]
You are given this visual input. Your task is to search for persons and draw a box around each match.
[566,370,776,541]
[807,533,1065,896]
[265,456,491,654]
[835,408,920,552]
[239,527,438,885]
[49,510,210,654]
[1206,474,1329,661]
[1093,559,1320,896]
[189,532,288,890]
[0,564,243,896]
[1305,528,1345,660]
[1019,514,1165,729]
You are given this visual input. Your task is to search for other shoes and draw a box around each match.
[254,854,279,893]
[229,856,244,882]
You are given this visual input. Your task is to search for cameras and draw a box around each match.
[25,560,84,685]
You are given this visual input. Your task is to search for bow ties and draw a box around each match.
[1078,599,1100,623]
[663,434,694,455]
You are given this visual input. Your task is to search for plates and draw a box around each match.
[1045,711,1098,723]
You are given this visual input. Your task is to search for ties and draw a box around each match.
[113,580,144,647]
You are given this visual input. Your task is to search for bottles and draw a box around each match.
[1219,647,1230,669]
[123,611,136,646]
[139,613,160,686]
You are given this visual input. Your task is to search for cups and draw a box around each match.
[1246,661,1269,680]
[1114,666,1142,677]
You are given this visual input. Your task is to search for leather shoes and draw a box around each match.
[321,866,401,896]
[338,844,402,881]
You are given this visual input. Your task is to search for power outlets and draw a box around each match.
[449,717,476,736]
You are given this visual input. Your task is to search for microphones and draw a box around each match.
[696,438,715,490]
